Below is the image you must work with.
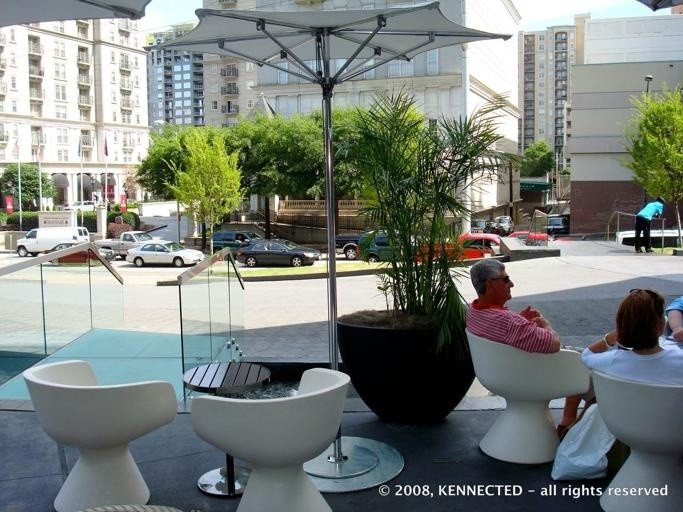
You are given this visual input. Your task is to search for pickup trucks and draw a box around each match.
[417,233,503,262]
[335,233,369,260]
[94,230,155,260]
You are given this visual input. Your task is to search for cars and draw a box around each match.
[236,239,323,266]
[506,231,572,241]
[126,241,205,266]
[64,201,95,211]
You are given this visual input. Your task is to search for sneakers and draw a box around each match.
[636,247,655,253]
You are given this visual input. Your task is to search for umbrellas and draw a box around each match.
[142,0,514,455]
[0,0,150,29]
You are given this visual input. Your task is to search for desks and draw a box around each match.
[182,361,271,499]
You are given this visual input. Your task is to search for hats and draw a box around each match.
[657,195,666,204]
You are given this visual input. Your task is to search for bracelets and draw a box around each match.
[604,333,614,349]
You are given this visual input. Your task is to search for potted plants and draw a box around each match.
[337,86,523,423]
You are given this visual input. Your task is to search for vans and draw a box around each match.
[358,228,419,261]
[12,225,90,258]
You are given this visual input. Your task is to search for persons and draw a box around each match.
[665,295,682,345]
[581,289,682,388]
[633,196,664,253]
[465,258,594,439]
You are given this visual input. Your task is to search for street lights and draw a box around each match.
[642,74,655,207]
[152,118,181,244]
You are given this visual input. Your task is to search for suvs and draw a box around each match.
[490,216,516,234]
[472,218,491,232]
[205,230,262,252]
[544,213,570,235]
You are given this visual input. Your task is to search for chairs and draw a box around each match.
[21,361,178,512]
[590,371,683,512]
[188,367,352,512]
[464,328,590,465]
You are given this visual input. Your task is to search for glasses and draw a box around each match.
[627,288,658,315]
[480,275,510,284]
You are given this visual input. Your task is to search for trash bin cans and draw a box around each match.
[138,203,143,216]
